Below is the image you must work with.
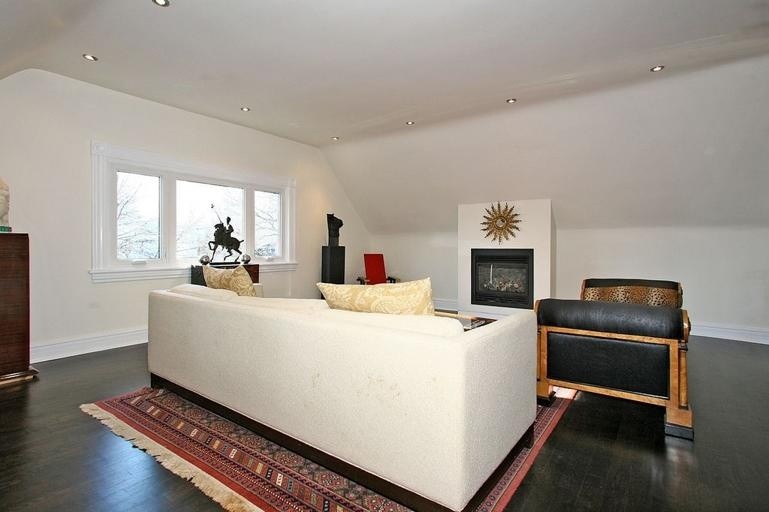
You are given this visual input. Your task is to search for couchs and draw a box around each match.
[147,281,536,511]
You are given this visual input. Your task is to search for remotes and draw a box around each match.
[464,319,485,330]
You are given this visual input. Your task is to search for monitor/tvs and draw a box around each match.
[471,248,534,309]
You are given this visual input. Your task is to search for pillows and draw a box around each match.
[315,276,434,318]
[204,265,254,296]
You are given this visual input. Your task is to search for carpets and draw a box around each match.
[79,386,577,510]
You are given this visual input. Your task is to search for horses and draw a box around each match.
[208,223,244,262]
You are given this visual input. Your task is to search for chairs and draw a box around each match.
[535,276,693,444]
[356,252,395,284]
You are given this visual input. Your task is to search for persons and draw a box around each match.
[221,217,234,252]
